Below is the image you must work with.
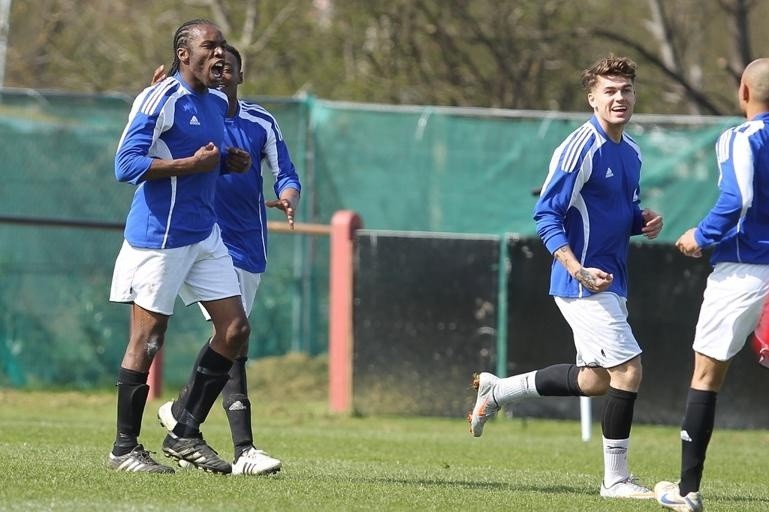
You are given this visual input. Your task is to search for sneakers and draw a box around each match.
[157,399,232,476]
[232,443,282,476]
[468,372,502,438]
[600,476,655,500]
[106,443,175,474]
[655,481,703,511]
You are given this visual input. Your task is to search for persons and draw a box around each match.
[654,56,768,511]
[150,46,301,475]
[106,20,251,475]
[468,53,665,500]
